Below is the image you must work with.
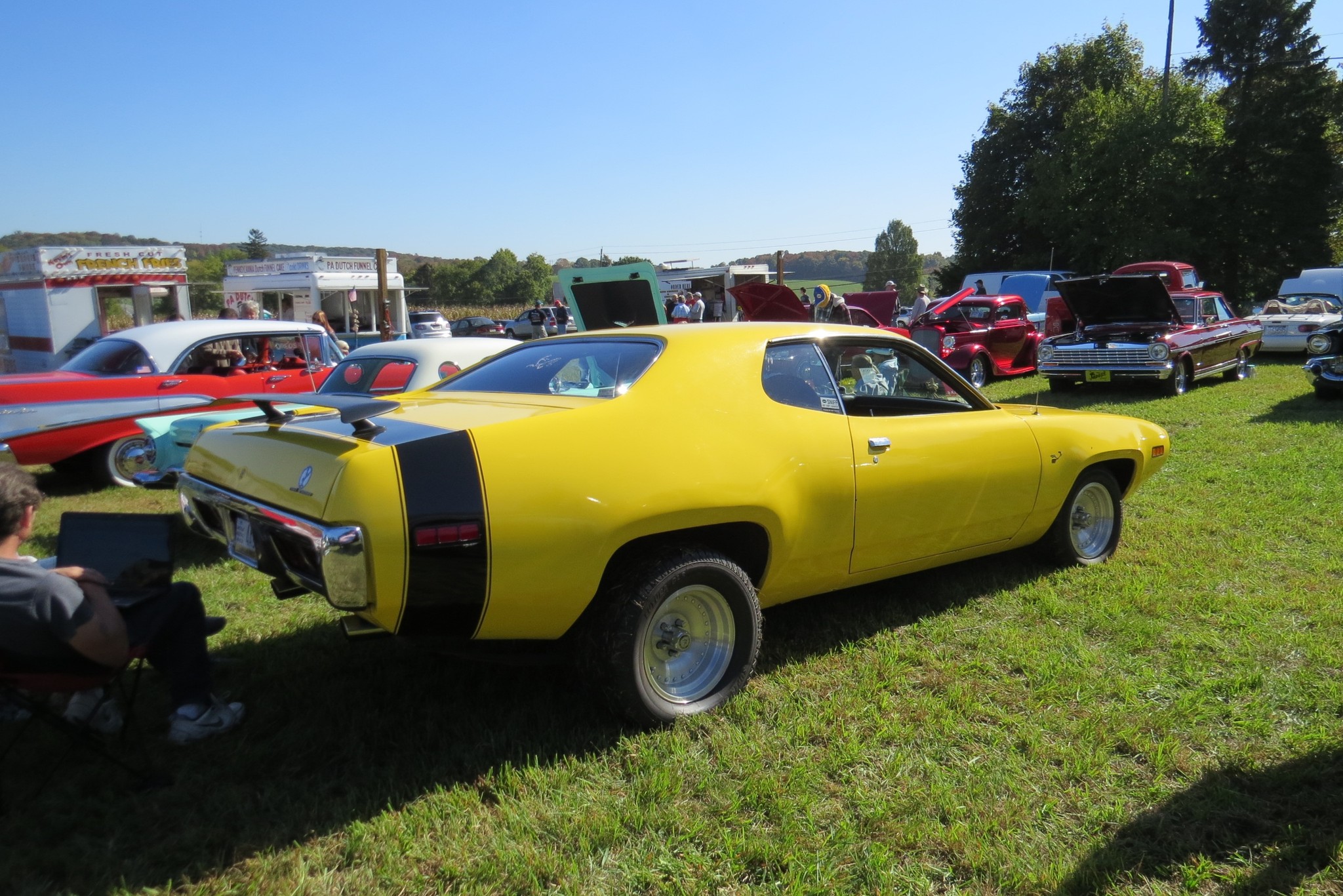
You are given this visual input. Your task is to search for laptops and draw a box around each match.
[51,511,178,610]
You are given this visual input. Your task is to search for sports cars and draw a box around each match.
[169,314,1172,729]
[1,319,355,489]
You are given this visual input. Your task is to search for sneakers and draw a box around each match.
[167,699,245,747]
[67,693,124,731]
[201,616,226,638]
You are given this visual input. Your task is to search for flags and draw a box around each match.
[348,286,357,302]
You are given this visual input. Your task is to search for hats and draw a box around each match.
[885,281,897,287]
[553,300,561,307]
[865,347,894,355]
[915,284,929,294]
[974,279,983,284]
[813,284,831,308]
[692,292,702,298]
[535,301,543,305]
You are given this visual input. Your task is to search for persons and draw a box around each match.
[974,280,986,295]
[528,300,548,340]
[685,292,695,308]
[553,300,569,335]
[678,290,683,295]
[671,295,690,323]
[667,294,679,322]
[578,357,590,382]
[0,463,244,744]
[850,348,899,395]
[911,284,930,320]
[884,281,901,319]
[311,311,338,343]
[800,287,811,305]
[168,300,260,374]
[809,285,854,385]
[689,292,705,322]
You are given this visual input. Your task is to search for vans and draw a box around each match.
[1110,262,1204,296]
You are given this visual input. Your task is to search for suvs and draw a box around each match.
[406,312,455,339]
[501,305,579,340]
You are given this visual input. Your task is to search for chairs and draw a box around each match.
[0,646,176,800]
[763,374,821,408]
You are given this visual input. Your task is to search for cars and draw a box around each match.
[135,263,673,488]
[1033,275,1266,397]
[1300,318,1342,394]
[729,280,1043,380]
[1240,268,1343,359]
[451,318,506,337]
